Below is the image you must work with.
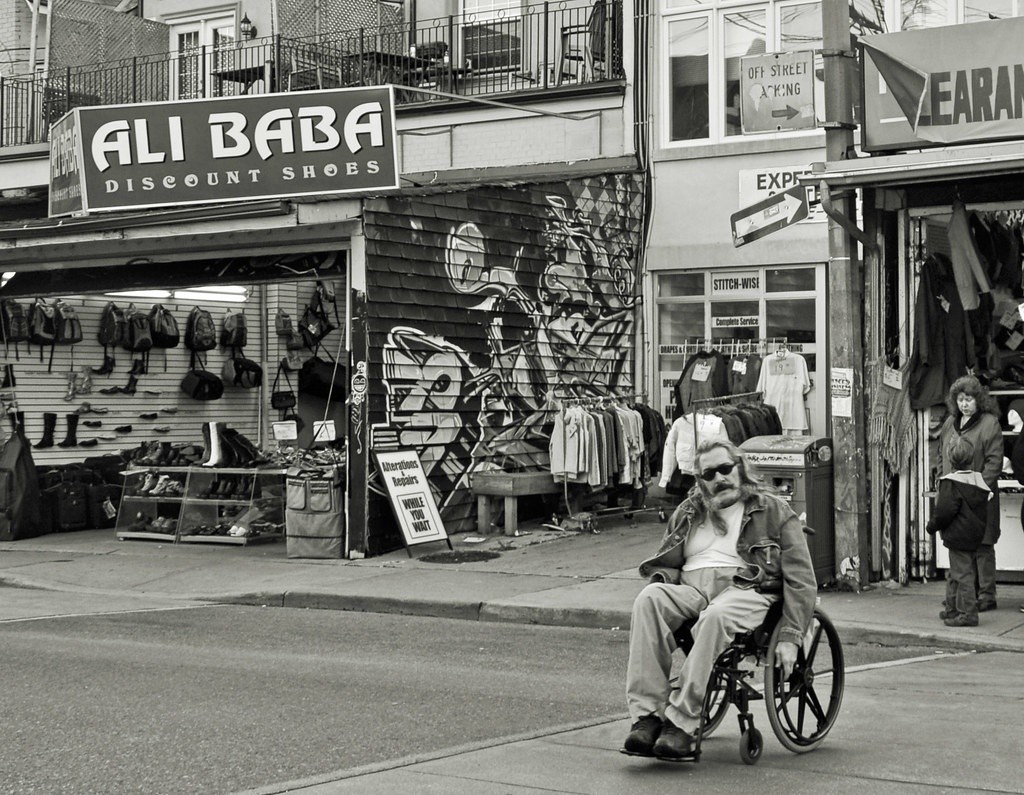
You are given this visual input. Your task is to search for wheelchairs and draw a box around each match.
[619,526,845,765]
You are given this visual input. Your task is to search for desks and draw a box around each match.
[345,51,440,102]
[209,65,265,98]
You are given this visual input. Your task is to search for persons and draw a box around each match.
[926,436,991,626]
[624,439,818,755]
[942,376,1003,612]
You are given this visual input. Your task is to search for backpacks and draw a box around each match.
[149,303,181,373]
[48,298,83,373]
[123,301,154,375]
[309,278,342,331]
[1,421,48,543]
[185,306,218,369]
[220,306,248,363]
[1,298,33,362]
[28,295,55,363]
[97,299,128,367]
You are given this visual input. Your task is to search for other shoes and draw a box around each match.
[1019,607,1024,613]
[0,354,279,538]
[980,602,997,611]
[943,616,980,628]
[939,609,959,620]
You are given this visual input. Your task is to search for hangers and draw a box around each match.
[560,392,648,411]
[695,392,764,415]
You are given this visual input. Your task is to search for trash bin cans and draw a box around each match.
[738,434,835,590]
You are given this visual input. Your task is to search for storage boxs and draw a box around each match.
[285,463,347,559]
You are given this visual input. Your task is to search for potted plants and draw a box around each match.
[429,39,450,69]
[416,42,433,71]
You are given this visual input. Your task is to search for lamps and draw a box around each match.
[240,13,257,43]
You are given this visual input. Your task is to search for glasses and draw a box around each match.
[694,461,739,481]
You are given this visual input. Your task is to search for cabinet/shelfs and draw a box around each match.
[923,389,1024,497]
[113,464,285,549]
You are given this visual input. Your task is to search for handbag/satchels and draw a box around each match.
[185,351,262,400]
[34,451,132,534]
[270,308,355,445]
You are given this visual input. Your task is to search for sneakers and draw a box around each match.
[623,706,662,753]
[653,718,693,758]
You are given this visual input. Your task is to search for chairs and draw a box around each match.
[288,22,610,105]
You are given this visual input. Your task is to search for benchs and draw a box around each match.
[473,471,582,536]
